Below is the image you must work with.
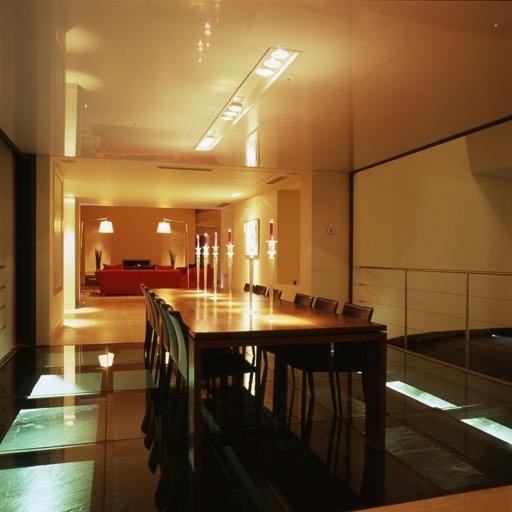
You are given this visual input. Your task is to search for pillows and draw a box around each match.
[103,263,122,270]
[156,264,174,270]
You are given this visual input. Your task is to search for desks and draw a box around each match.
[150,286,388,473]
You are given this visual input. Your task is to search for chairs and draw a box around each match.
[307,303,374,421]
[140,283,259,473]
[291,293,314,307]
[292,297,342,419]
[244,285,282,299]
[186,267,213,289]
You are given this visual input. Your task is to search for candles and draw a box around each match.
[269,222,274,240]
[228,232,232,242]
[196,234,217,247]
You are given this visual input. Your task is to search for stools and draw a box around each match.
[86,275,97,287]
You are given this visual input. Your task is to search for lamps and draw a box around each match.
[157,217,188,235]
[81,213,114,236]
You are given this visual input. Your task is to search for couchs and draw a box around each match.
[95,270,180,295]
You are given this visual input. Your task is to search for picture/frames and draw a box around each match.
[244,218,259,257]
